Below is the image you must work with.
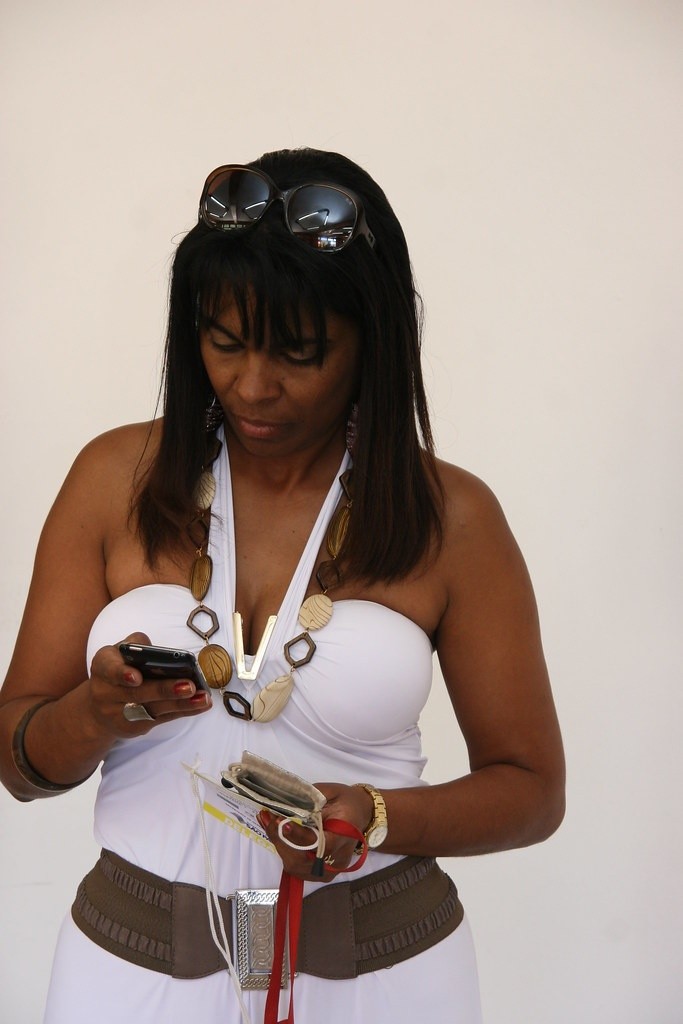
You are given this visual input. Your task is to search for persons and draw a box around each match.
[0,147,565,1024]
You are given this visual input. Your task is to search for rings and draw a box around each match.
[123,702,156,722]
[325,856,335,865]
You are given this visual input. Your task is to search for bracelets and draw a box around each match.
[11,697,98,792]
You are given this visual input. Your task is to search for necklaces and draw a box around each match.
[187,436,352,724]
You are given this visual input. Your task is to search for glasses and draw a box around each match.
[198,166,376,253]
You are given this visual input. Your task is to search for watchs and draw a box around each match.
[351,782,388,856]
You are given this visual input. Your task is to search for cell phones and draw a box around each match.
[119,643,212,698]
